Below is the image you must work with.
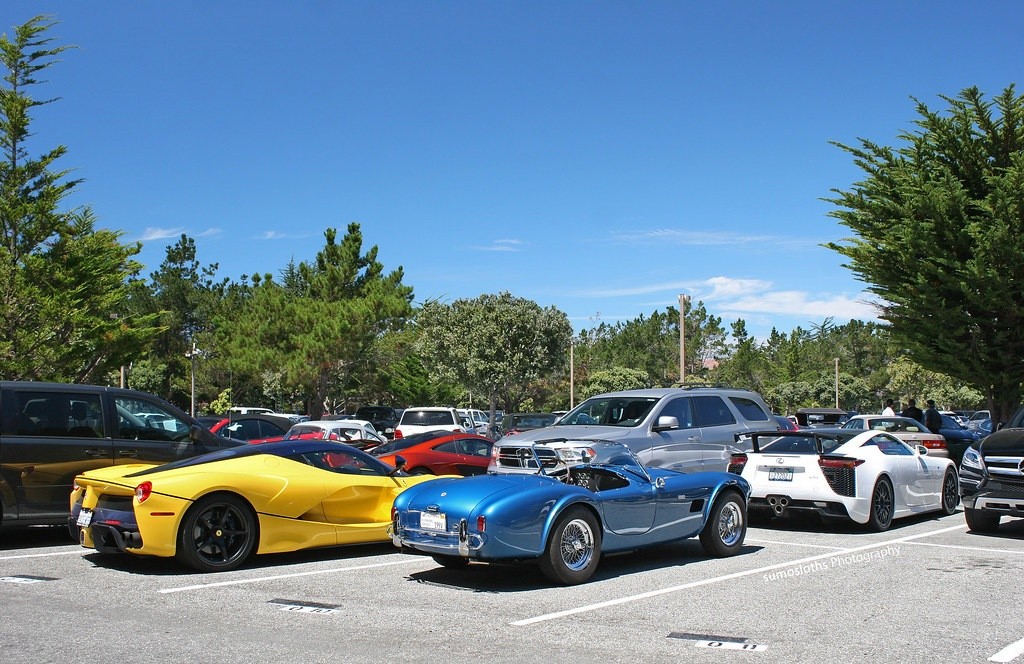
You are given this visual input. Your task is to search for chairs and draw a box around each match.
[541,421,549,426]
[906,427,919,432]
[308,453,330,470]
[873,426,886,430]
[467,441,476,454]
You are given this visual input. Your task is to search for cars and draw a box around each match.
[769,408,991,435]
[837,414,952,461]
[24,398,600,473]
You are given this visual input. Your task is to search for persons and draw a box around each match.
[925,400,942,433]
[881,399,896,427]
[902,399,923,426]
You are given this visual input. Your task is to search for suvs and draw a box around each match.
[0,381,266,549]
[956,400,1024,532]
[487,382,780,484]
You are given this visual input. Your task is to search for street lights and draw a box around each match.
[832,356,842,411]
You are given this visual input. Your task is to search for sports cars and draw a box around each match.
[353,429,500,479]
[65,436,470,574]
[388,437,753,586]
[724,427,961,531]
